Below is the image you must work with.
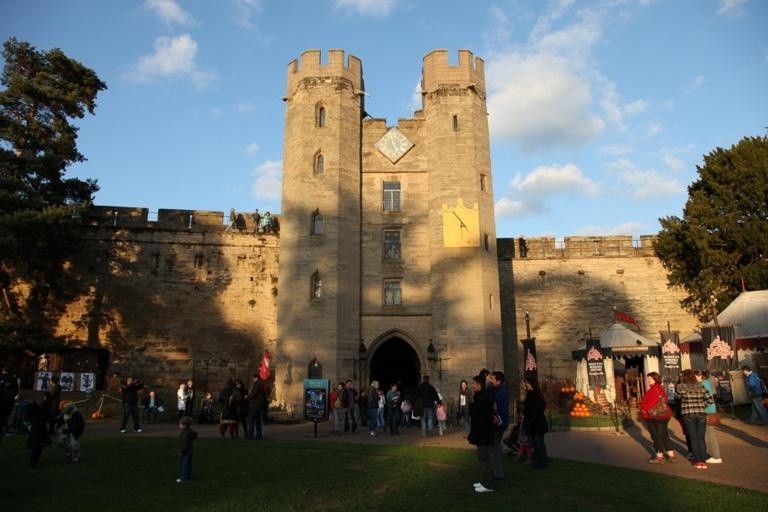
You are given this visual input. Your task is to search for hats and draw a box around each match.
[59,400,75,414]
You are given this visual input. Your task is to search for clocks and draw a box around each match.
[370,124,416,165]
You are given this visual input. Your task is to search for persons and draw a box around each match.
[200,392,214,422]
[120,378,144,432]
[176,380,195,425]
[141,391,164,423]
[456,380,472,423]
[0,364,86,466]
[219,373,268,439]
[640,369,722,469]
[232,208,274,232]
[741,365,768,424]
[176,416,197,483]
[467,369,548,494]
[329,375,450,436]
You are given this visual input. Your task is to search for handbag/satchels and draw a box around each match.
[648,397,668,416]
[705,412,720,426]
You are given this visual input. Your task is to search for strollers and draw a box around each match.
[502,410,536,462]
[7,398,43,437]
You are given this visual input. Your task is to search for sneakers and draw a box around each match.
[649,458,664,465]
[473,483,494,493]
[686,452,707,469]
[705,457,722,463]
[667,457,676,463]
